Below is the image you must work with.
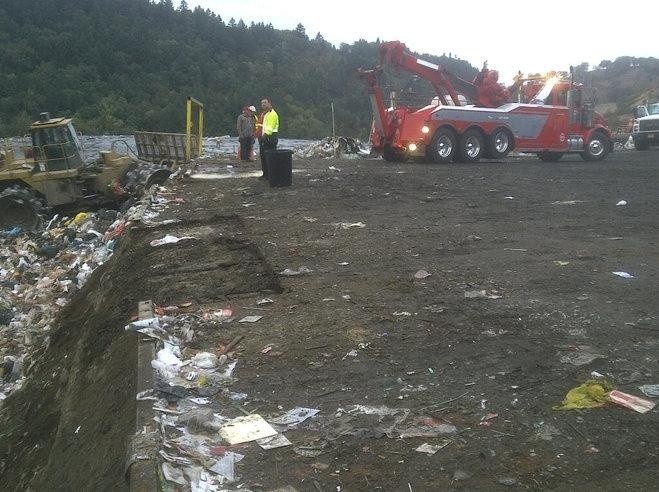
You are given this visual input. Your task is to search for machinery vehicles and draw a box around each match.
[360,40,614,164]
[0,111,199,232]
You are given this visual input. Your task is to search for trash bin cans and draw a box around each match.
[265,149,294,187]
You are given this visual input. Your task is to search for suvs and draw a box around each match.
[630,103,659,150]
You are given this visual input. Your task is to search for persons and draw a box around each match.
[236,96,279,181]
